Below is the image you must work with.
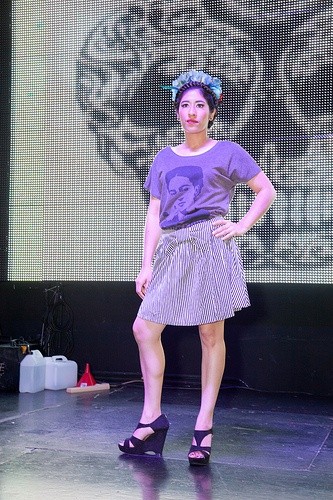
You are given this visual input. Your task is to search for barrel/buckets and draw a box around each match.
[44,355,77,390]
[19,349,45,393]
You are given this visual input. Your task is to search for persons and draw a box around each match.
[118,69,277,466]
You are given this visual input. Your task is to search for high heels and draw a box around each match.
[188,428,213,465]
[118,414,169,458]
